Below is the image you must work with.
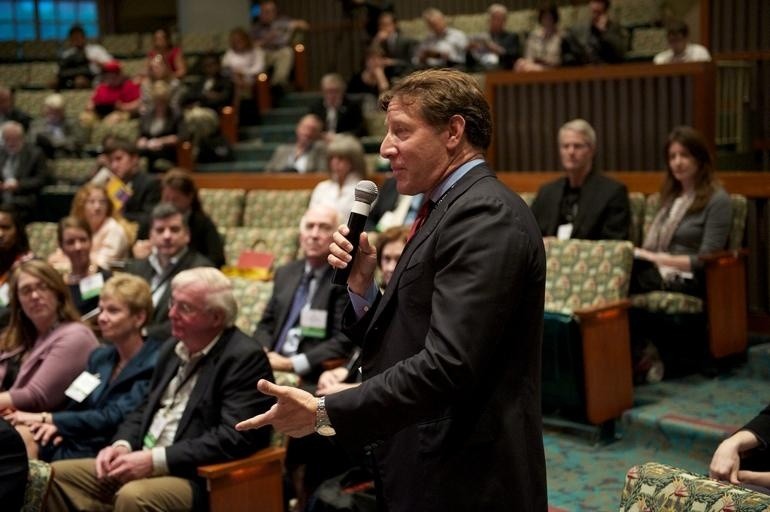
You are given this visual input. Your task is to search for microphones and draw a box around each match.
[331,180,379,286]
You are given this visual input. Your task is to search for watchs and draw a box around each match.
[313,399,337,439]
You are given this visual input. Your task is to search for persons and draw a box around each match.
[0,3,310,172]
[355,3,631,111]
[652,18,714,66]
[2,136,423,510]
[263,73,368,173]
[709,401,769,493]
[236,66,549,510]
[621,123,734,386]
[530,117,633,246]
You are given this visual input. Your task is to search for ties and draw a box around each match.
[274,271,313,352]
[407,199,431,242]
